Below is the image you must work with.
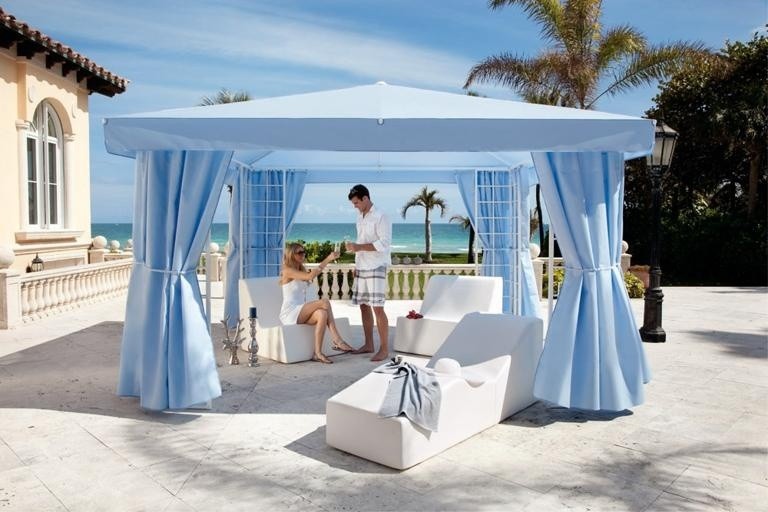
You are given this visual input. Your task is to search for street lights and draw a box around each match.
[638,107,680,343]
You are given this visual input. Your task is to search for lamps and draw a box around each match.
[31,253,43,271]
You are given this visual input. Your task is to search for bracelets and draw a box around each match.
[317,266,324,272]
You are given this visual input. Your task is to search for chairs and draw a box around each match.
[393,274,504,357]
[238,275,351,365]
[325,314,543,472]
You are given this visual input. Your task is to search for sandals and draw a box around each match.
[312,352,334,364]
[330,340,354,353]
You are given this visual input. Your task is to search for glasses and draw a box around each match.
[295,251,308,256]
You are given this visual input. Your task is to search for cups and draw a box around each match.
[335,241,341,256]
[344,235,353,253]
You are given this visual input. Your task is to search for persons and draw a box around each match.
[338,183,393,361]
[277,241,358,365]
